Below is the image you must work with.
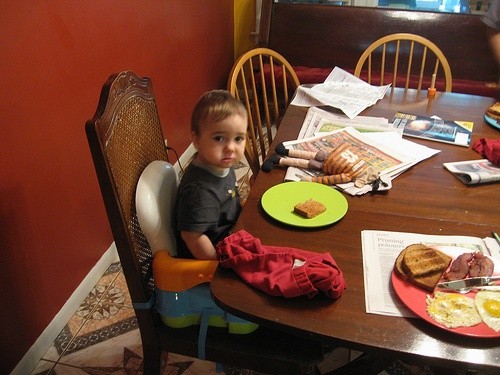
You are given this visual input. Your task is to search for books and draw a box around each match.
[394,111,473,147]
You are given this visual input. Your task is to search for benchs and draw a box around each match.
[248,0,500,117]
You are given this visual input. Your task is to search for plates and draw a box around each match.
[260,181,349,228]
[484,113,500,130]
[390,245,500,339]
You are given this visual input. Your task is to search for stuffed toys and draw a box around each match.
[260,143,387,192]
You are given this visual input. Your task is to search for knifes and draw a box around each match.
[438,276,500,289]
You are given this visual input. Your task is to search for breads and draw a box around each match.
[294,200,326,219]
[486,101,500,119]
[401,247,447,279]
[394,243,452,292]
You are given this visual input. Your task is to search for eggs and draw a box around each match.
[475,290,500,333]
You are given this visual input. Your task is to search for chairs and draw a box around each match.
[227,46,301,180]
[354,32,453,97]
[85,69,325,375]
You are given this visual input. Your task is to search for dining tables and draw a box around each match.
[206,83,500,375]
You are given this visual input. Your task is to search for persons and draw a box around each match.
[480,0,500,67]
[171,90,249,261]
